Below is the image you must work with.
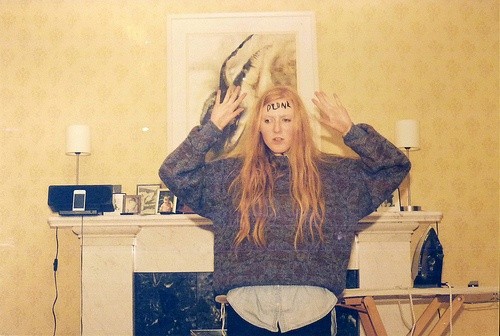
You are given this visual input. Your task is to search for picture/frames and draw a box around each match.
[113,193,126,211]
[122,195,141,214]
[155,189,177,214]
[137,183,160,215]
[376,188,400,213]
[166,12,322,159]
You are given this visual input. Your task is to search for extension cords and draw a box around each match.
[468,280,478,288]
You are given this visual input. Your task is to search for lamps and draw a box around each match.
[66,126,90,184]
[396,119,421,211]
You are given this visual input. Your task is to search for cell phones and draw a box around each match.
[72,190,86,212]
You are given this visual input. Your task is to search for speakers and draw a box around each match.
[48,184,121,215]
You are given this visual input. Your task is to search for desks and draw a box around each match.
[341,286,500,336]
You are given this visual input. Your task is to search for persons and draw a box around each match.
[157,87,412,336]
